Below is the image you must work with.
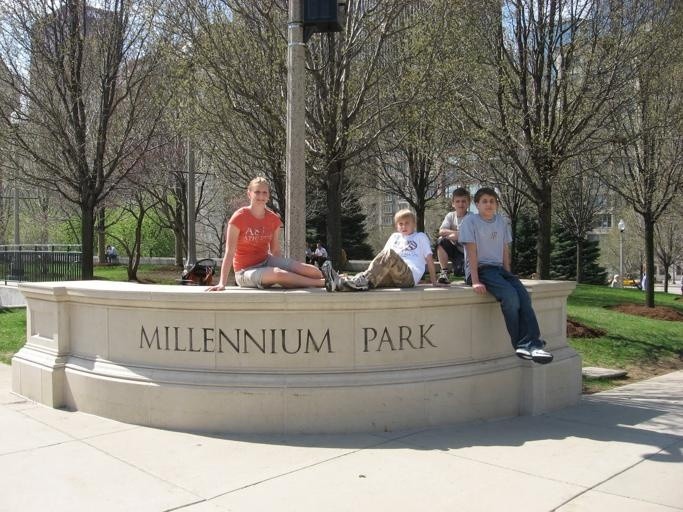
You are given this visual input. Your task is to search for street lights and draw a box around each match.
[182,43,198,266]
[617,219,626,287]
[9,112,22,261]
[494,187,504,215]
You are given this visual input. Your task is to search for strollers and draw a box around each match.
[176,258,216,286]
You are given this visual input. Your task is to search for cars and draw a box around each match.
[623,278,640,287]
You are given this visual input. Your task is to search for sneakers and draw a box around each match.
[437,270,451,284]
[342,273,369,291]
[515,347,554,364]
[321,260,337,293]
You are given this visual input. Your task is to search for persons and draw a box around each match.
[309,242,328,267]
[202,175,354,294]
[187,266,213,286]
[679,272,683,296]
[456,187,553,366]
[103,245,117,263]
[632,263,645,292]
[319,207,452,294]
[610,273,622,289]
[304,241,312,264]
[433,187,476,285]
